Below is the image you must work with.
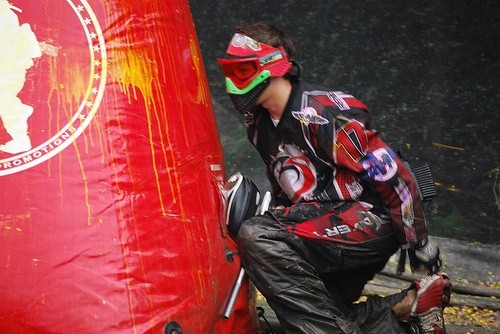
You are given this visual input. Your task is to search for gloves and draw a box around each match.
[408,232,444,278]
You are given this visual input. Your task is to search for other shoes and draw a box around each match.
[402,271,453,334]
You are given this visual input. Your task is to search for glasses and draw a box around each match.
[217,50,285,90]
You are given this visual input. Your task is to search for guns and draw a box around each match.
[219,169,271,320]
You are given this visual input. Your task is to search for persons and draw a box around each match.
[216,19,455,334]
